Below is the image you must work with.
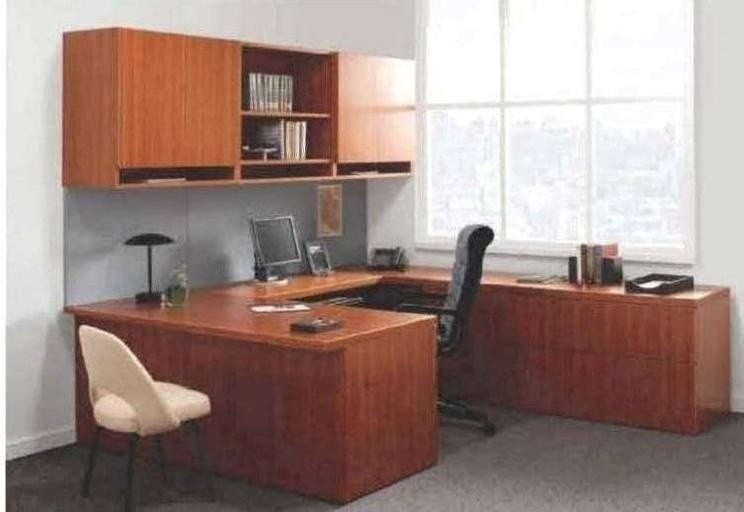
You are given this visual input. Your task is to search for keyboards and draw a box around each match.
[315,296,363,306]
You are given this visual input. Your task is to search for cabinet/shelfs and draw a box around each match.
[243,43,335,184]
[62,31,242,189]
[329,52,415,180]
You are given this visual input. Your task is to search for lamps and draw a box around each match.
[123,232,175,301]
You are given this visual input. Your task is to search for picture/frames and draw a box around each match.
[302,238,331,274]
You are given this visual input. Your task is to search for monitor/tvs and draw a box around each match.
[248,214,301,284]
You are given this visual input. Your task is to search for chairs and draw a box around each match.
[76,324,218,512]
[382,222,499,440]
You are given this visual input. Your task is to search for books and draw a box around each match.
[251,117,309,160]
[242,69,294,113]
[288,314,343,334]
[516,270,561,285]
[565,242,623,285]
